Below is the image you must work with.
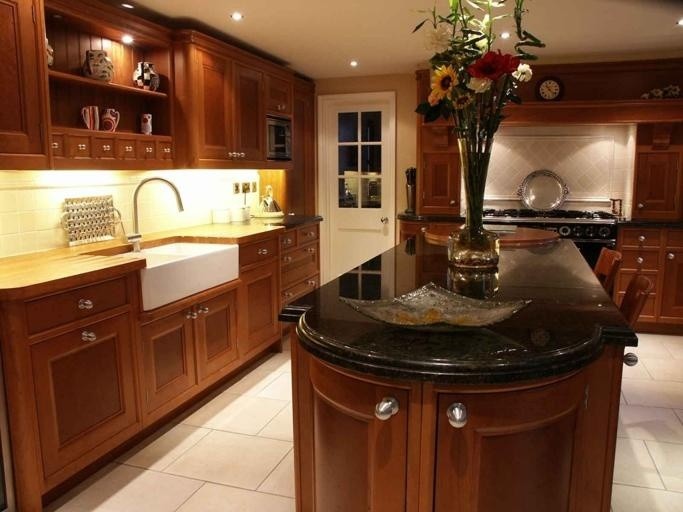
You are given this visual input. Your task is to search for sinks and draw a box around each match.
[114,241,240,313]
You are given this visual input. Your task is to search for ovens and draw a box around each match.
[547,224,618,302]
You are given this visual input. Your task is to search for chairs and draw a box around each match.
[619,275,654,329]
[593,247,622,294]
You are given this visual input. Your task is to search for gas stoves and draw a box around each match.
[482,205,616,221]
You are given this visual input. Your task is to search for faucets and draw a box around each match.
[129,176,184,235]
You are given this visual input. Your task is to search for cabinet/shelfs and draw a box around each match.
[25,274,138,495]
[0,0,53,171]
[174,32,266,168]
[238,237,279,357]
[141,288,238,415]
[625,121,683,222]
[43,1,182,170]
[280,222,320,352]
[416,127,469,216]
[308,355,585,511]
[265,62,297,169]
[658,229,683,324]
[415,226,447,289]
[612,227,661,322]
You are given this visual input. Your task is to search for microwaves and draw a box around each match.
[266,116,293,160]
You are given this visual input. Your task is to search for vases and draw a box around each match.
[448,138,501,269]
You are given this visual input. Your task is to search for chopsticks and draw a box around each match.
[406,167,416,185]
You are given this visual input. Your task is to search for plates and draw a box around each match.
[337,282,535,336]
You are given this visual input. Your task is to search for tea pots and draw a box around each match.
[253,185,285,217]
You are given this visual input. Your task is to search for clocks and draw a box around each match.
[535,76,565,101]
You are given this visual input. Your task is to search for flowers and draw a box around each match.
[409,0,546,241]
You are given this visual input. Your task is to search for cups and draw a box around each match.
[80,105,100,130]
[140,113,153,136]
[101,108,120,132]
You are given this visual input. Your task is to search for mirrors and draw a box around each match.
[516,170,569,213]
